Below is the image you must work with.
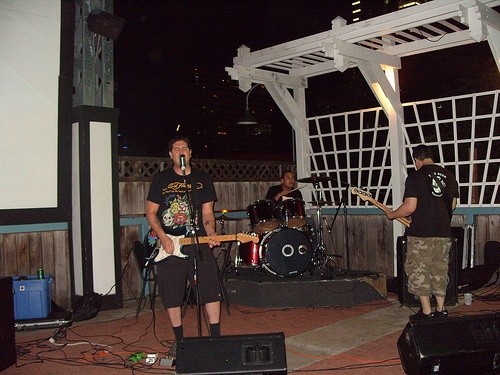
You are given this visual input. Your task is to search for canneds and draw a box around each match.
[37,269,44,280]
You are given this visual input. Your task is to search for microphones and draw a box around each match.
[179,154,186,170]
[323,217,331,233]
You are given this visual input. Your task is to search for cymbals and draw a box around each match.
[215,214,243,221]
[295,176,330,183]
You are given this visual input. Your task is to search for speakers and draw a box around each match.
[175,332,287,375]
[397,312,500,375]
[0,275,18,372]
[396,236,458,307]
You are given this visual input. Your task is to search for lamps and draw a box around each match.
[238,83,261,124]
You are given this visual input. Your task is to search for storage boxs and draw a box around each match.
[13,275,54,320]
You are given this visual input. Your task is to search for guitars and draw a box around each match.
[351,186,412,228]
[149,230,260,264]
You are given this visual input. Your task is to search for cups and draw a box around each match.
[463,293,473,306]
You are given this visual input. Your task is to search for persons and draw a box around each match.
[146,136,223,339]
[386,145,459,320]
[265,171,303,203]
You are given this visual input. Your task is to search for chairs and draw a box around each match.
[133,241,194,318]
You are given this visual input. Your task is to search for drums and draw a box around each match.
[238,232,271,266]
[276,198,307,228]
[258,227,312,279]
[246,198,283,235]
[291,222,311,236]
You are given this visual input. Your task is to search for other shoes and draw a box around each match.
[432,308,448,318]
[409,308,435,320]
[168,342,177,357]
